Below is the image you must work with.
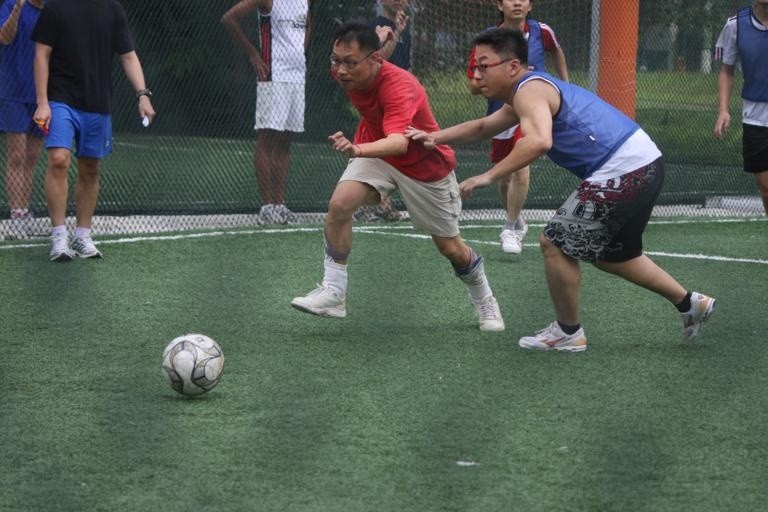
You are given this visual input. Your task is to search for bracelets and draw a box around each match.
[355,144,361,157]
[136,88,152,97]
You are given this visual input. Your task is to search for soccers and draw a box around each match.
[160,333,225,395]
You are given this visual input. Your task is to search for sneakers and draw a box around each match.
[513,218,529,248]
[376,205,407,223]
[277,205,304,224]
[518,321,587,353]
[256,205,290,228]
[70,236,105,260]
[49,230,73,263]
[3,219,27,241]
[290,284,348,319]
[500,228,522,254]
[26,216,53,237]
[468,292,506,333]
[679,291,717,346]
[352,207,383,224]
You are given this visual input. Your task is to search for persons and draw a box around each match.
[220,0,312,229]
[717,1,768,218]
[351,0,411,224]
[291,24,506,333]
[405,27,717,352]
[468,0,569,253]
[30,2,155,263]
[0,0,44,240]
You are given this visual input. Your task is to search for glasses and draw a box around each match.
[468,57,516,73]
[329,50,375,68]
